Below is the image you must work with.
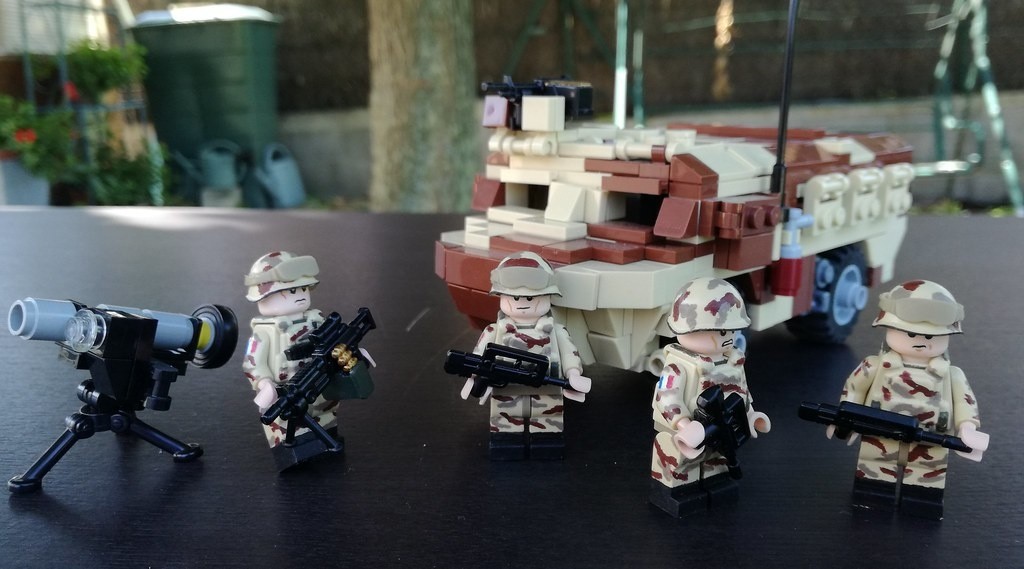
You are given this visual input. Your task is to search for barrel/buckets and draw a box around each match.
[130,15,286,211]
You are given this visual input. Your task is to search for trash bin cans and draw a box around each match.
[129,2,277,210]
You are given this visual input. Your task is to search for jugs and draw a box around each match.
[248,138,307,211]
[166,136,246,190]
